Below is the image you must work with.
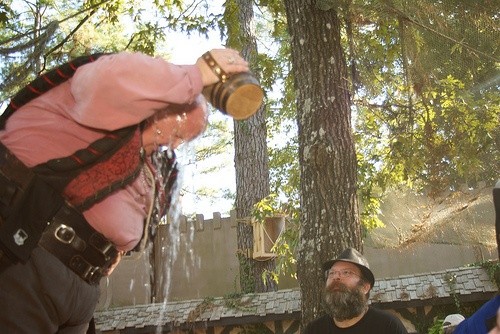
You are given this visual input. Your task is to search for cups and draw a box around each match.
[201,51,264,120]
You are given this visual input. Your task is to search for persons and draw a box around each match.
[0,48,249,334]
[302,247,408,334]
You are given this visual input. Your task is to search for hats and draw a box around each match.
[324,246,375,289]
[442,314,465,328]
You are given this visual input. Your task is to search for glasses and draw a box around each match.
[325,270,366,279]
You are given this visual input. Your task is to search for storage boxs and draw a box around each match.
[251,213,286,262]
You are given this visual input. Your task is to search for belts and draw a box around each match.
[0,144,125,287]
